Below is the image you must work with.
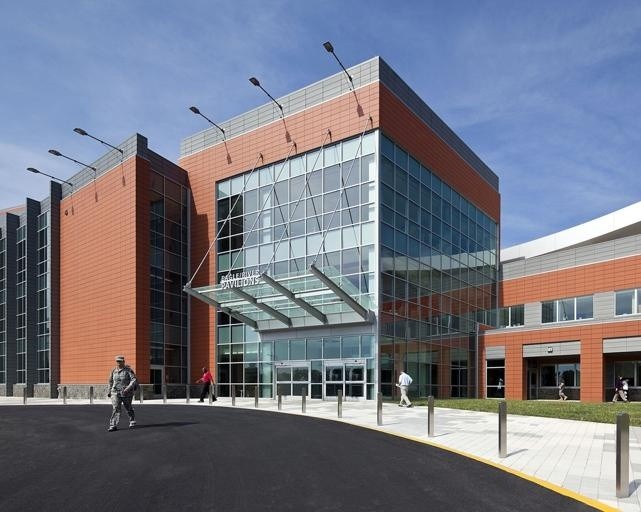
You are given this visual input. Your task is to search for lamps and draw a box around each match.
[26,127,124,186]
[188,41,353,133]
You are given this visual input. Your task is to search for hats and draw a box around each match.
[115,356,125,363]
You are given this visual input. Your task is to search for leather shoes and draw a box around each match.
[129,423,135,429]
[108,427,117,431]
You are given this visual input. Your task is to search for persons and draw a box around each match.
[394,367,414,409]
[621,377,632,402]
[107,354,139,431]
[195,366,218,405]
[555,376,568,401]
[612,375,630,404]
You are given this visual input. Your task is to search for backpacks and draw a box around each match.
[126,368,137,392]
